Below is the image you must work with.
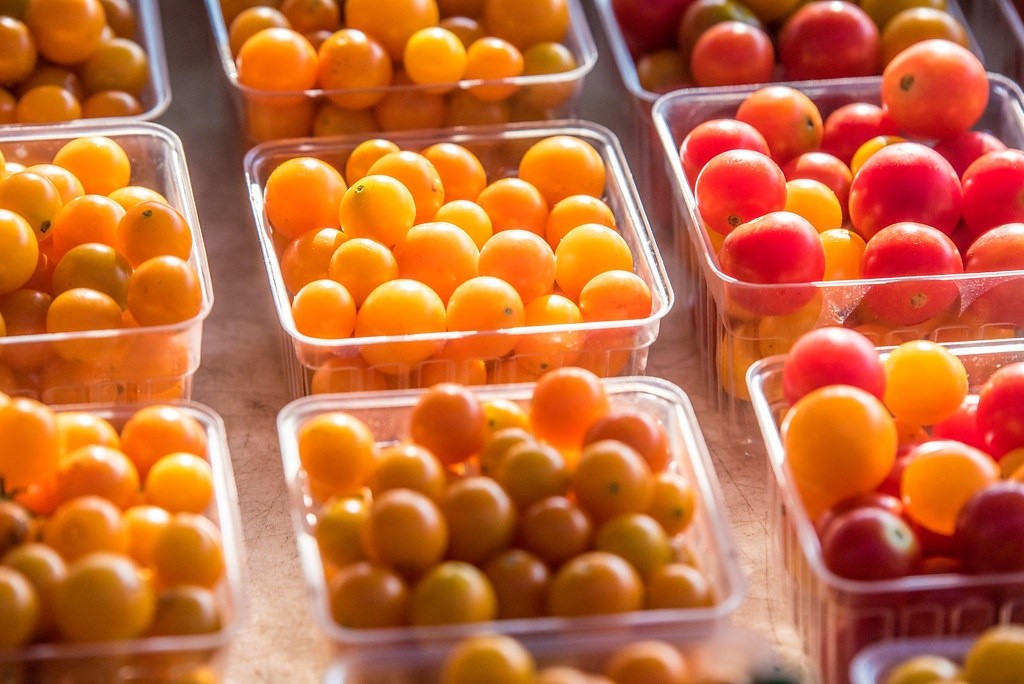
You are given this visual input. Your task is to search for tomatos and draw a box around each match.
[0,0,1024,684]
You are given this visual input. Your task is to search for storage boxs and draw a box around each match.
[0,0,1024,683]
